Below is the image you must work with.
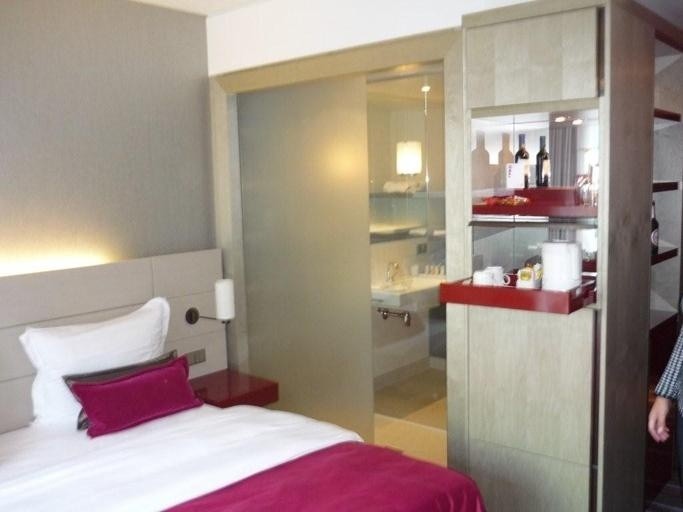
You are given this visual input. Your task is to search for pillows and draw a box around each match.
[19,297,171,429]
[68,353,205,439]
[63,348,178,431]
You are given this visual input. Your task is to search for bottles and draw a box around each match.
[514,134,528,186]
[537,135,550,186]
[650,202,659,254]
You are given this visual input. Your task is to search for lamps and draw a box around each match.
[396,140,423,176]
[185,277,236,328]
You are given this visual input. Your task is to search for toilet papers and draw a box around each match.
[542,238,584,294]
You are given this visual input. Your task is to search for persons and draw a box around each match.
[647,320,683,491]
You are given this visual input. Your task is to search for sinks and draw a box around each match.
[370,274,446,313]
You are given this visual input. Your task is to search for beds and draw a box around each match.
[0,247,484,512]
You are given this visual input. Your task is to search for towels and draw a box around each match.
[383,180,424,194]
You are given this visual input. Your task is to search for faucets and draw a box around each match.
[385,261,399,283]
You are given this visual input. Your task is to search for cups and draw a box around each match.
[580,184,591,208]
[591,186,598,207]
[487,266,510,285]
[473,270,494,285]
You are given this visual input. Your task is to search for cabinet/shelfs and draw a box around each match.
[438,105,683,512]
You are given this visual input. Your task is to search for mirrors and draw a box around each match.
[366,72,446,245]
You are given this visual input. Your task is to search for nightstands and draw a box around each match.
[189,366,279,408]
